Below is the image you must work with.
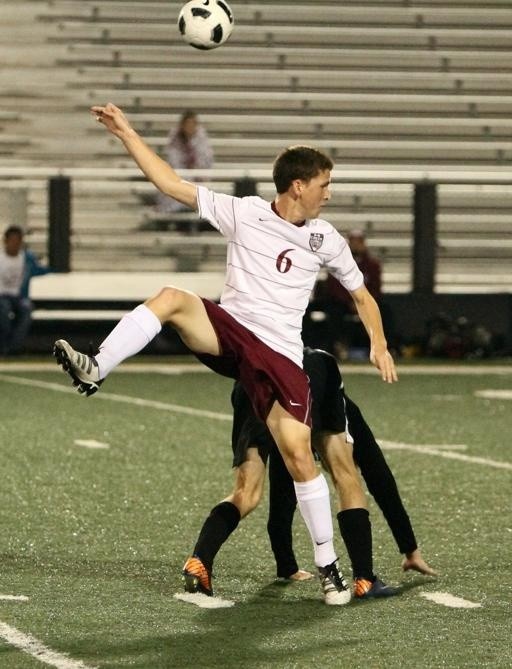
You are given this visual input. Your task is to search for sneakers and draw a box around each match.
[317,558,351,606]
[354,576,404,598]
[53,339,105,398]
[182,555,216,597]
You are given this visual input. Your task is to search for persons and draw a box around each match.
[163,107,214,176]
[323,229,384,364]
[0,224,52,359]
[179,344,442,600]
[49,103,401,608]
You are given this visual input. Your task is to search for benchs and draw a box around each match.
[0,1,511,288]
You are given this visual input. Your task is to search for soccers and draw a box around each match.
[178,0,234,50]
